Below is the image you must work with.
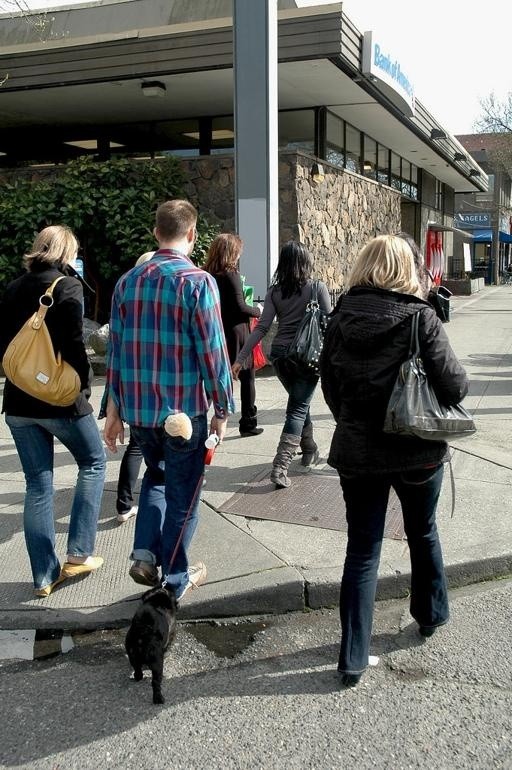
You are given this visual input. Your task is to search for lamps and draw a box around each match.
[430,127,481,179]
[310,164,326,185]
[363,160,372,170]
[141,81,166,98]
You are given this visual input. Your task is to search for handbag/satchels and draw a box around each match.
[384,357,477,440]
[2,311,82,408]
[286,308,331,377]
[250,317,266,370]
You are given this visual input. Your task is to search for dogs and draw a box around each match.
[124,581,182,704]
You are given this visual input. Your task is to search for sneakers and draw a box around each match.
[174,559,208,602]
[61,556,105,578]
[202,479,207,487]
[35,569,66,597]
[128,560,163,585]
[116,505,139,523]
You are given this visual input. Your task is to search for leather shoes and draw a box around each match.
[341,674,361,684]
[419,626,435,637]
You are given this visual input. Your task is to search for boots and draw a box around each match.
[239,406,264,437]
[300,422,319,467]
[270,431,303,488]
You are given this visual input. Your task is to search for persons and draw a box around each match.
[95,199,235,607]
[0,222,108,600]
[113,250,156,524]
[199,232,266,439]
[230,238,334,491]
[315,232,470,688]
